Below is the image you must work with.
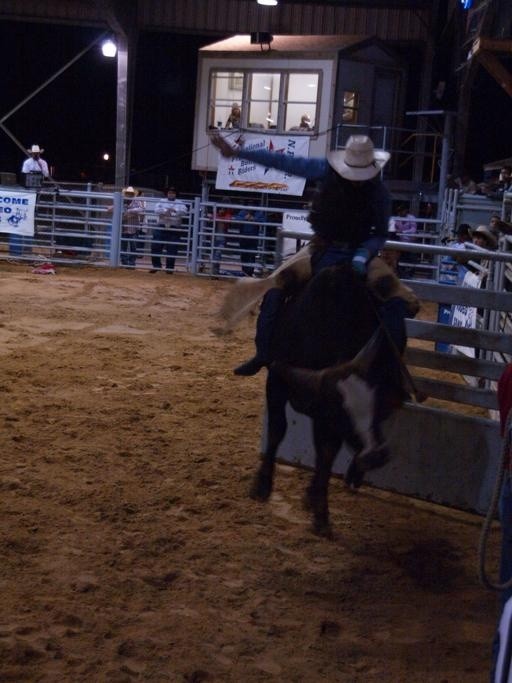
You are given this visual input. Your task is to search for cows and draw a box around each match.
[248,238,423,538]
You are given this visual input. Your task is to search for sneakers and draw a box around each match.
[148,266,175,274]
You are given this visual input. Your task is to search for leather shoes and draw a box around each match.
[235,353,266,378]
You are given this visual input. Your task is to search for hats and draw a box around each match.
[302,113,311,125]
[231,102,241,112]
[324,132,393,182]
[122,187,138,196]
[26,145,44,155]
[467,225,499,251]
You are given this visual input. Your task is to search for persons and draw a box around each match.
[209,132,421,377]
[22,143,49,183]
[289,112,313,132]
[225,102,240,128]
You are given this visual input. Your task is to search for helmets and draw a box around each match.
[166,185,178,195]
